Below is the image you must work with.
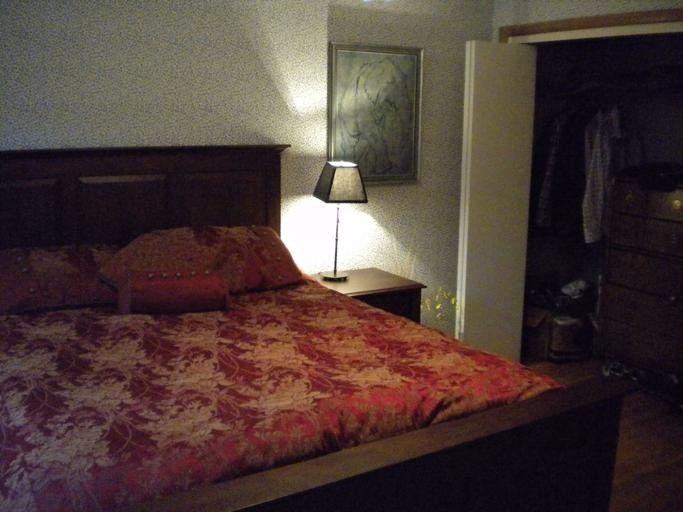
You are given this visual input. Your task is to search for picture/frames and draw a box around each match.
[327,40,425,186]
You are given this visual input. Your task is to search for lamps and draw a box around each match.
[312,160,368,281]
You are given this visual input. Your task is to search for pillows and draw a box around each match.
[119,276,239,314]
[98,225,303,297]
[0,242,120,311]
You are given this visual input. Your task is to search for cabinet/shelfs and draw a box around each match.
[599,174,683,381]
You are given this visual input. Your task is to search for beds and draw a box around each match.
[0,143,634,511]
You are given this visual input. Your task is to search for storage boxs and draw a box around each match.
[522,307,604,366]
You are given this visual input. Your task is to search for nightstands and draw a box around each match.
[309,269,427,328]
